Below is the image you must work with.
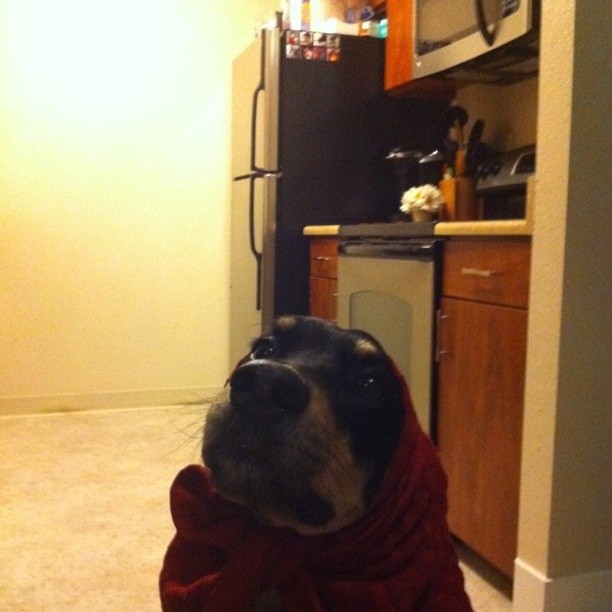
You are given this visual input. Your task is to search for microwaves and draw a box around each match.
[411,0,541,89]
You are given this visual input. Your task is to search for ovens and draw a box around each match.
[337,220,445,446]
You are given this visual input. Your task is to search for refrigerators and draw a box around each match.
[228,28,454,381]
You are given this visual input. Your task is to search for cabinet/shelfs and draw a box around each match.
[432,240,529,581]
[305,235,339,324]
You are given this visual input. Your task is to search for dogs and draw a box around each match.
[160,316,473,612]
